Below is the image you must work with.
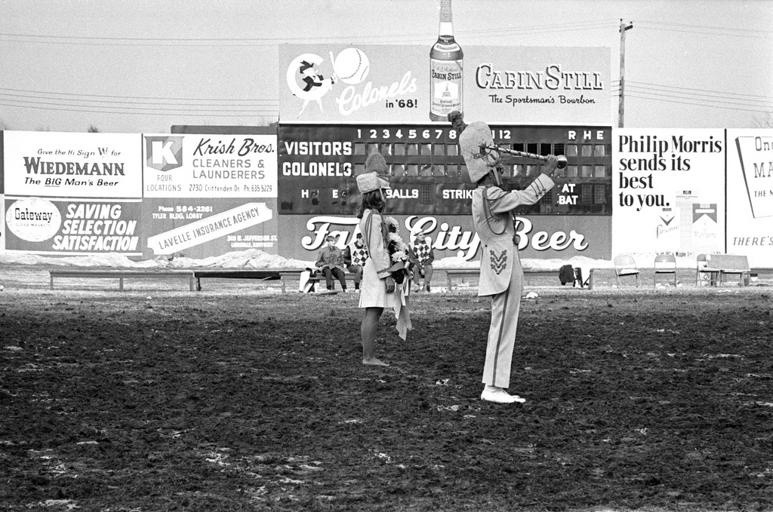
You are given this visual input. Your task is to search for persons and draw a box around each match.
[349,184,401,368]
[345,233,368,293]
[407,229,435,294]
[315,235,348,294]
[453,154,561,404]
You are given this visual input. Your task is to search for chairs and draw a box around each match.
[613,251,677,287]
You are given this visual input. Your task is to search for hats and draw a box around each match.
[458,122,504,183]
[355,171,391,194]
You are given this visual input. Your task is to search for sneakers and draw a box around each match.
[481,389,527,404]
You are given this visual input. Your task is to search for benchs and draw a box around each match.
[696,251,750,285]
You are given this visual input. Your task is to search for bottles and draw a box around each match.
[429,0,464,122]
